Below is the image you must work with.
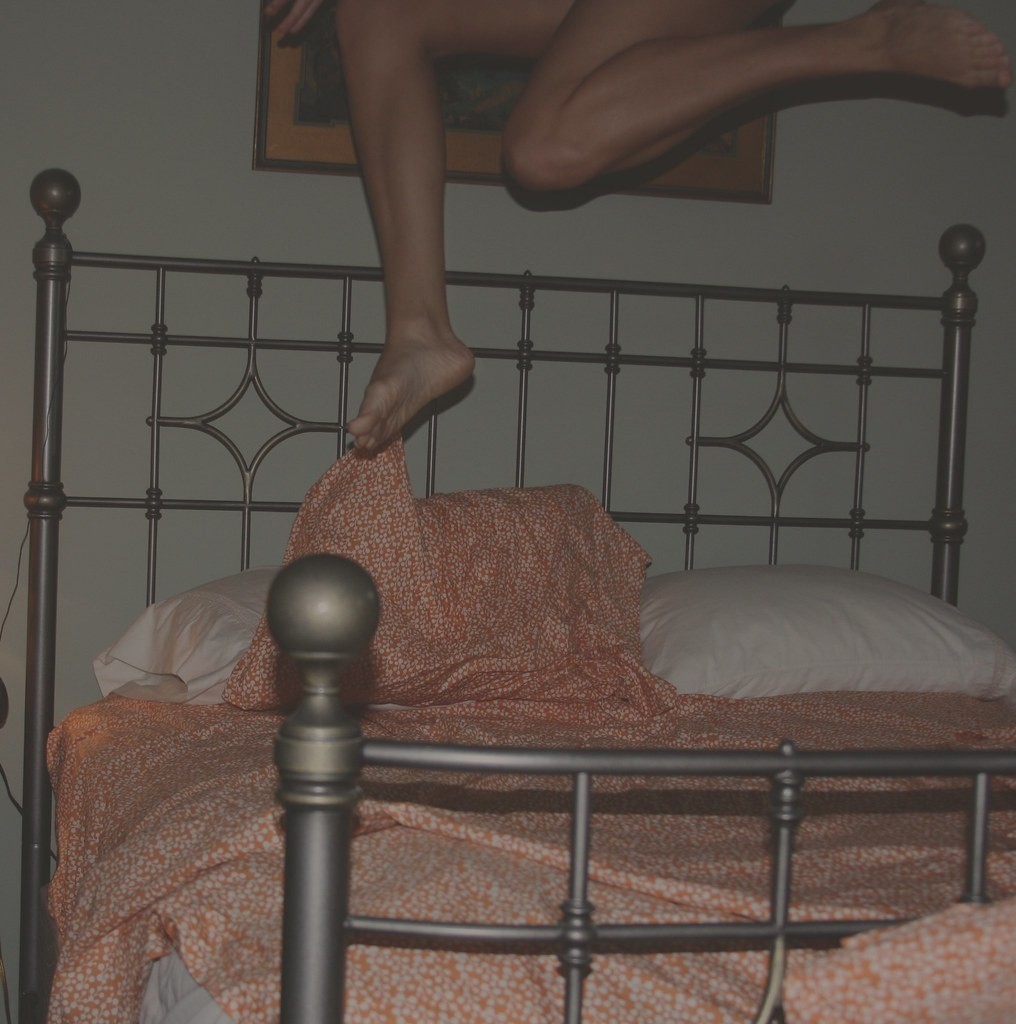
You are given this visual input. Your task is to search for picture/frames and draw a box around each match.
[252,0,781,203]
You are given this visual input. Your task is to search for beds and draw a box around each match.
[18,169,1016,1024]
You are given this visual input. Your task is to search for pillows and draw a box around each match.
[636,574,1016,700]
[222,431,676,711]
[94,563,282,707]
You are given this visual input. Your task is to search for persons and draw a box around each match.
[265,0,1010,456]
